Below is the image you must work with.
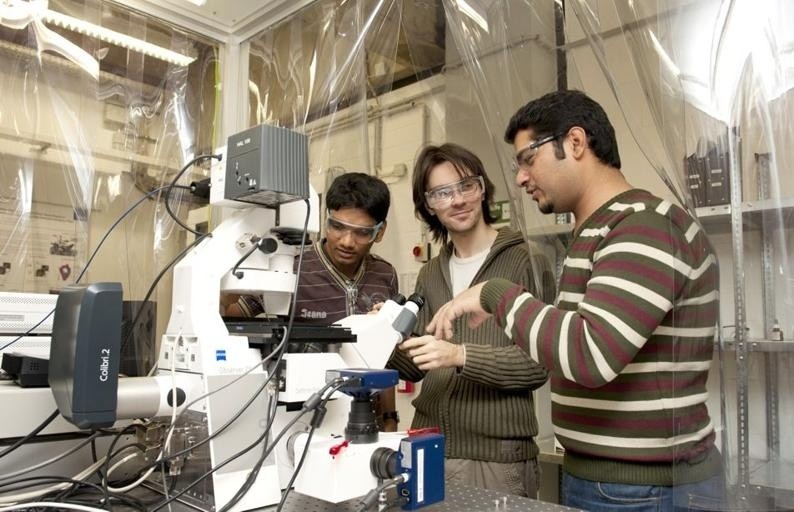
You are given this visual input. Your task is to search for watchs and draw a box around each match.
[381,410,400,423]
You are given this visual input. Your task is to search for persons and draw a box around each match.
[425,89,728,512]
[365,141,559,499]
[225,173,401,433]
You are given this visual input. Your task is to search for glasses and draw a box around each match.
[513,131,569,170]
[323,208,383,245]
[425,175,486,210]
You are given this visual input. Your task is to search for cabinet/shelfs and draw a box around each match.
[527,195,794,510]
